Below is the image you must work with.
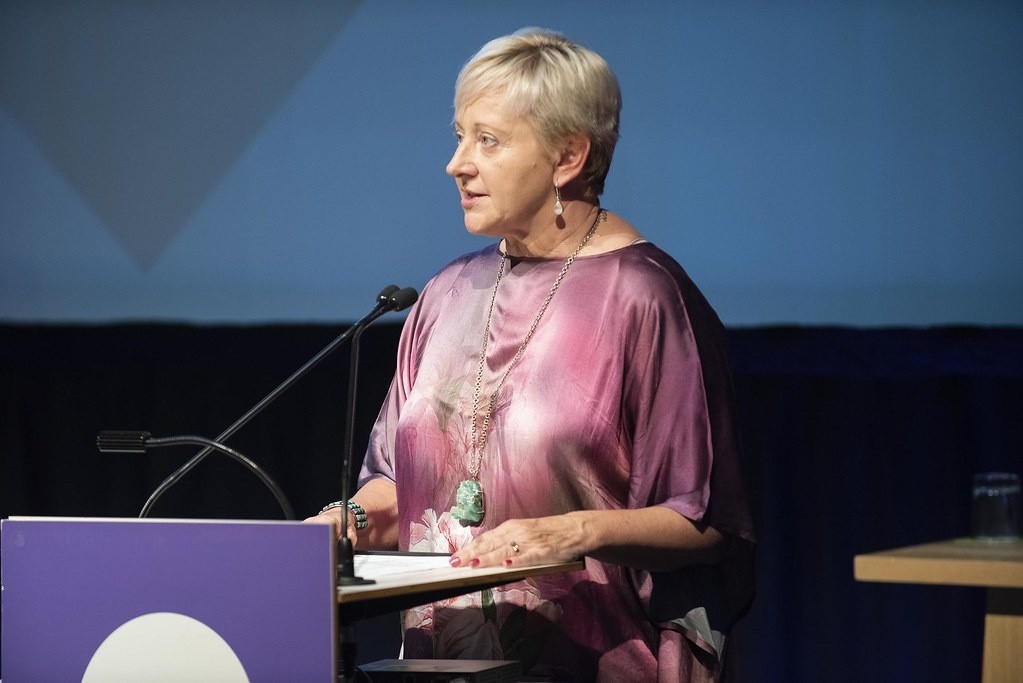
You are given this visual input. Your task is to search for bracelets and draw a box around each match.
[318,500,368,530]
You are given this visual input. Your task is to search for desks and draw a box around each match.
[854,539,1023,683]
[338,551,586,683]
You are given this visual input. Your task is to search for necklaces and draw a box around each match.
[449,209,602,523]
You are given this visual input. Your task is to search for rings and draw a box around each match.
[509,542,520,556]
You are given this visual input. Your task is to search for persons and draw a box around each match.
[303,26,755,683]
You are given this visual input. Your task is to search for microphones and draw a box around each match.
[95,283,416,587]
